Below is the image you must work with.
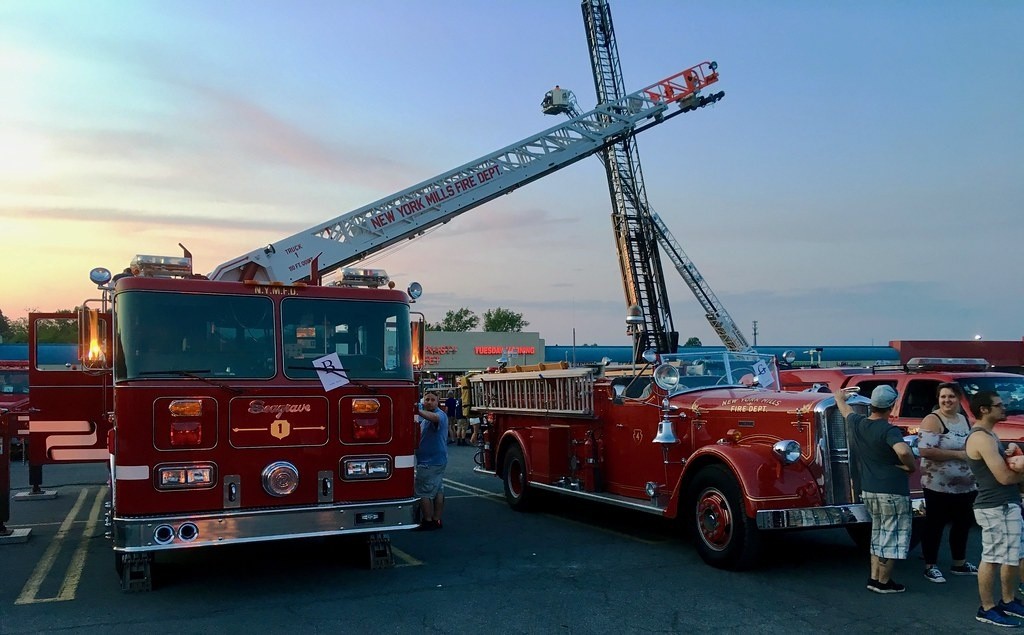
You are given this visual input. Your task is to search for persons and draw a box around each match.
[833,384,917,593]
[964,391,1024,627]
[445,390,482,446]
[418,390,449,531]
[1004,443,1024,473]
[917,381,978,583]
[1017,492,1024,595]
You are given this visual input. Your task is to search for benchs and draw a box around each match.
[611,374,737,401]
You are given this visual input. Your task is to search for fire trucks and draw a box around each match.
[778,355,1024,500]
[472,352,880,566]
[29,56,729,586]
[0,360,30,459]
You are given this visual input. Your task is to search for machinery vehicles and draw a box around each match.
[578,0,693,376]
[542,85,761,363]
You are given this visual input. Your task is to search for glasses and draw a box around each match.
[425,392,438,397]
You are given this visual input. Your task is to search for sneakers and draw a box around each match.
[1019,583,1024,594]
[951,562,979,577]
[975,605,1020,629]
[924,566,946,583]
[996,598,1024,618]
[868,578,905,594]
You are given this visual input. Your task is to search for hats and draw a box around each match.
[983,402,1005,407]
[870,385,900,407]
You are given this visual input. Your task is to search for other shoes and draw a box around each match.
[421,518,442,529]
